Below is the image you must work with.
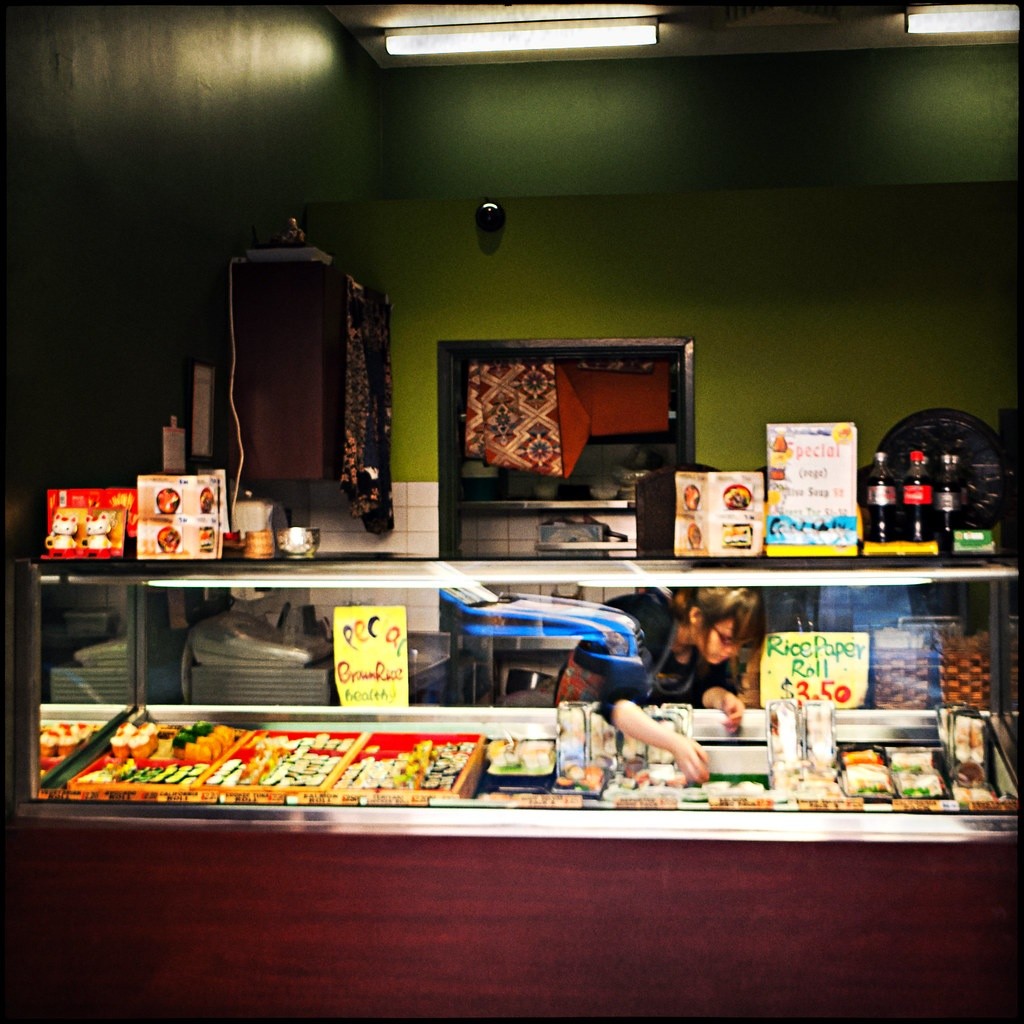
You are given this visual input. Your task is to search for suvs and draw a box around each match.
[436,585,652,707]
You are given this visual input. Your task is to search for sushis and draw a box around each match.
[35,702,1002,812]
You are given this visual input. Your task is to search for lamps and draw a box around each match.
[902,4,1024,36]
[381,13,661,57]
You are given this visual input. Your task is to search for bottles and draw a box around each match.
[867,452,966,553]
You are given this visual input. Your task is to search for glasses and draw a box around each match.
[711,625,750,648]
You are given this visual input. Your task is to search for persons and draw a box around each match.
[553,584,760,786]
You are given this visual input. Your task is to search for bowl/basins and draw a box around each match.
[274,526,321,559]
